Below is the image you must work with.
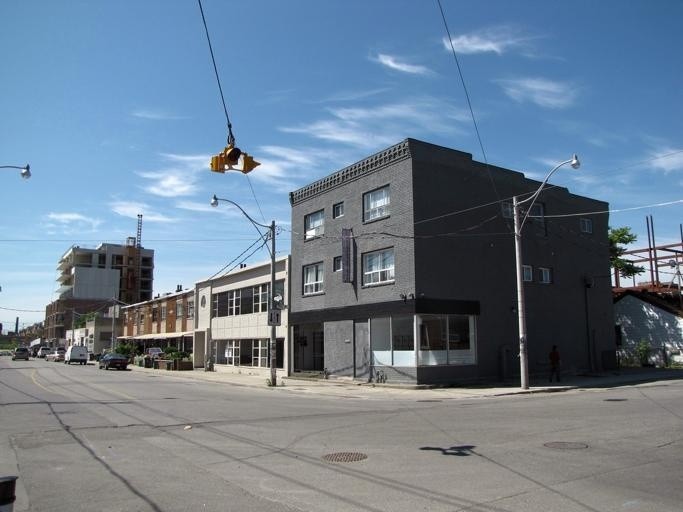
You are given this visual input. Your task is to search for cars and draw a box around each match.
[99,353,128,370]
[11,347,29,360]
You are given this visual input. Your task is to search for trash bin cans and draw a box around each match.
[206,359,214,371]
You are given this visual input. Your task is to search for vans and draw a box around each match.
[37,346,88,365]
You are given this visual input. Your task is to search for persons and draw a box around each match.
[548,344,561,382]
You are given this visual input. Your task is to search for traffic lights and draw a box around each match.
[210,145,261,174]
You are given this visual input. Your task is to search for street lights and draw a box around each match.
[512,154,580,388]
[0,164,31,179]
[211,194,277,385]
